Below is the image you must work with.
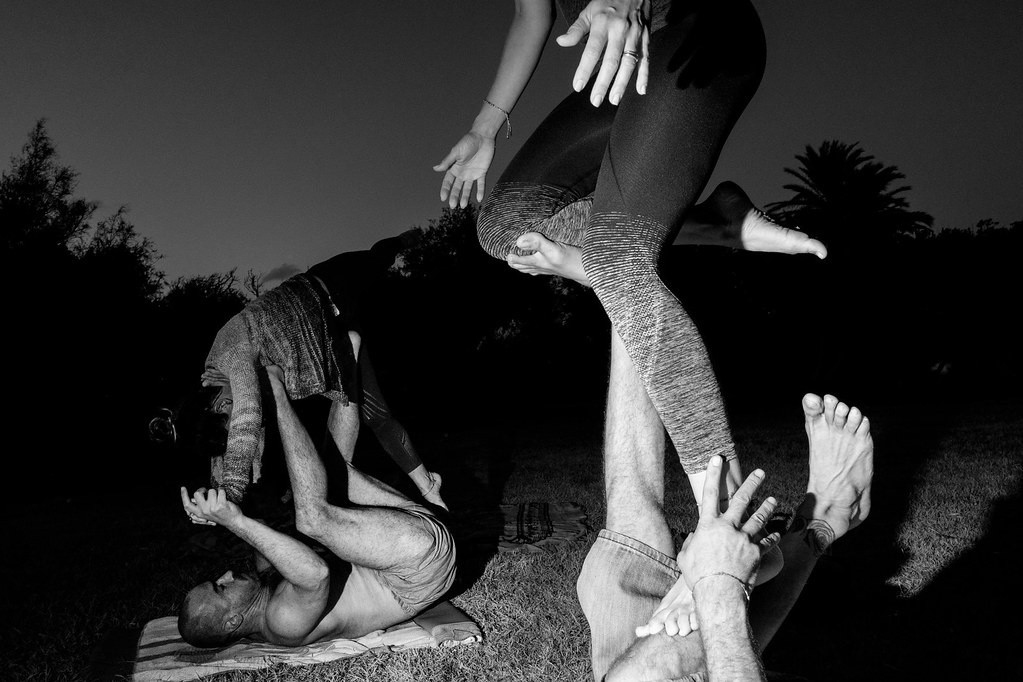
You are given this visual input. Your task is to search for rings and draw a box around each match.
[623,51,639,63]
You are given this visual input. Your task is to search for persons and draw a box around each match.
[147,228,449,518]
[431,1,830,637]
[505,230,875,682]
[177,330,458,649]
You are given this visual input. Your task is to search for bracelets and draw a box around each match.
[482,99,513,139]
[693,572,751,610]
